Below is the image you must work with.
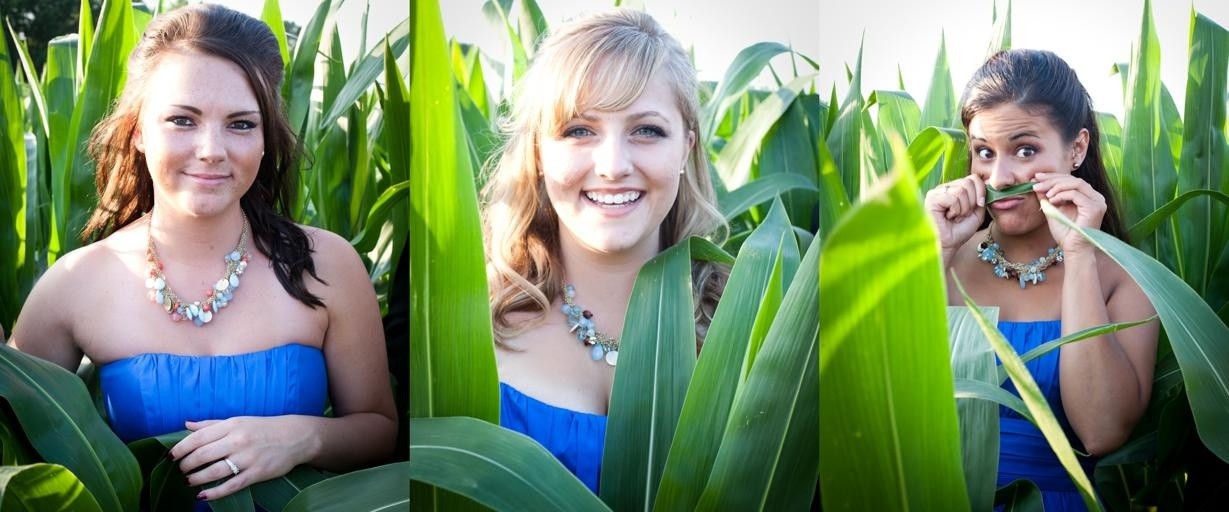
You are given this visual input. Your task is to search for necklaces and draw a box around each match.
[145,206,253,326]
[554,278,622,366]
[976,220,1064,289]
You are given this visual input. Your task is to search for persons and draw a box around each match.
[481,8,736,493]
[924,48,1162,511]
[1,4,401,502]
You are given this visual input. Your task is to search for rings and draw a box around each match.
[226,457,240,475]
[941,183,950,193]
